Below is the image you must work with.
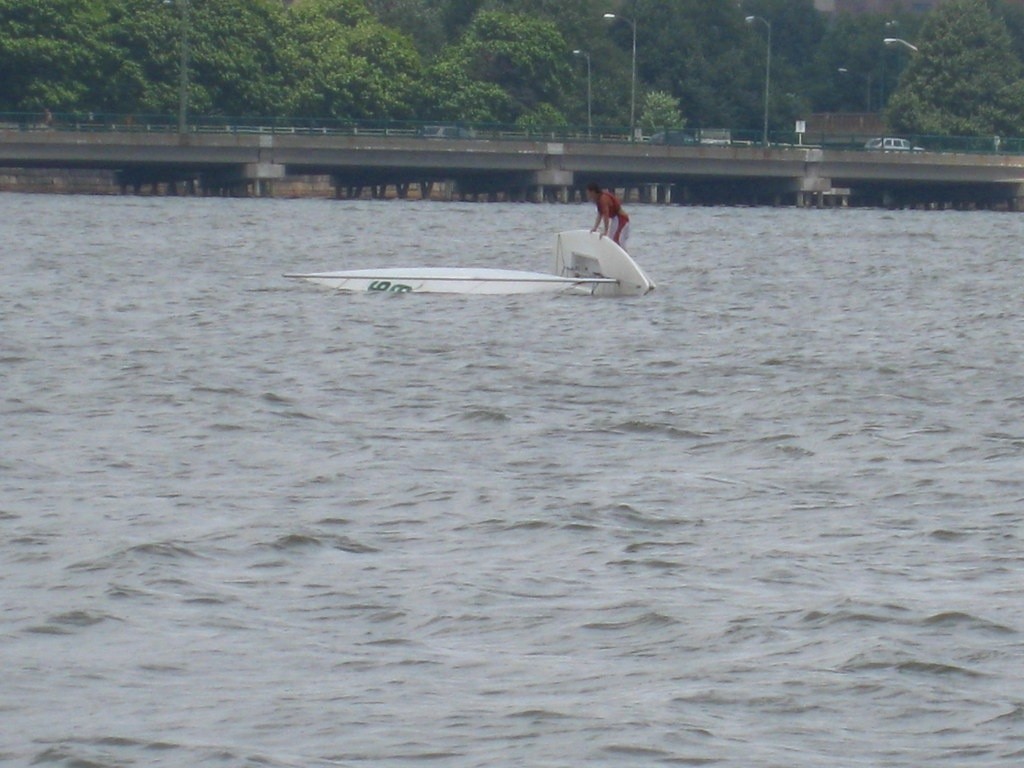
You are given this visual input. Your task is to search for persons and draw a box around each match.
[586,181,630,252]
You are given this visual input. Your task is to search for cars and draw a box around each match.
[650,132,696,142]
[865,138,925,154]
[419,126,472,140]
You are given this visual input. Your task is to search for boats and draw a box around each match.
[281,229,657,296]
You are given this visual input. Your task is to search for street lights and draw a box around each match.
[573,49,592,133]
[745,16,772,146]
[604,14,636,141]
